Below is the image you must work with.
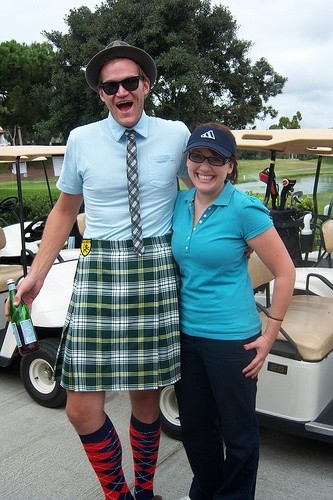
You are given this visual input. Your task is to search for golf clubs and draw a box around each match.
[291,191,303,206]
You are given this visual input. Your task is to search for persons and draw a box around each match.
[5,41,253,500]
[171,122,296,500]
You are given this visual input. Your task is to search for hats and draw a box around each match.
[184,130,238,158]
[85,39,156,94]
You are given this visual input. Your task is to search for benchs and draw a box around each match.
[243,217,333,423]
[0,226,34,330]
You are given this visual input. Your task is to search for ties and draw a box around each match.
[123,129,144,257]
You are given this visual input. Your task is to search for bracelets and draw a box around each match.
[269,316,283,321]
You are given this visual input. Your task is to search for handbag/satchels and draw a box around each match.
[270,209,302,268]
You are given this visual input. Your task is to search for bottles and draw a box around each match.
[6,278,40,355]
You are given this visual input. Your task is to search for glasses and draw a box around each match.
[99,76,144,95]
[188,152,232,166]
